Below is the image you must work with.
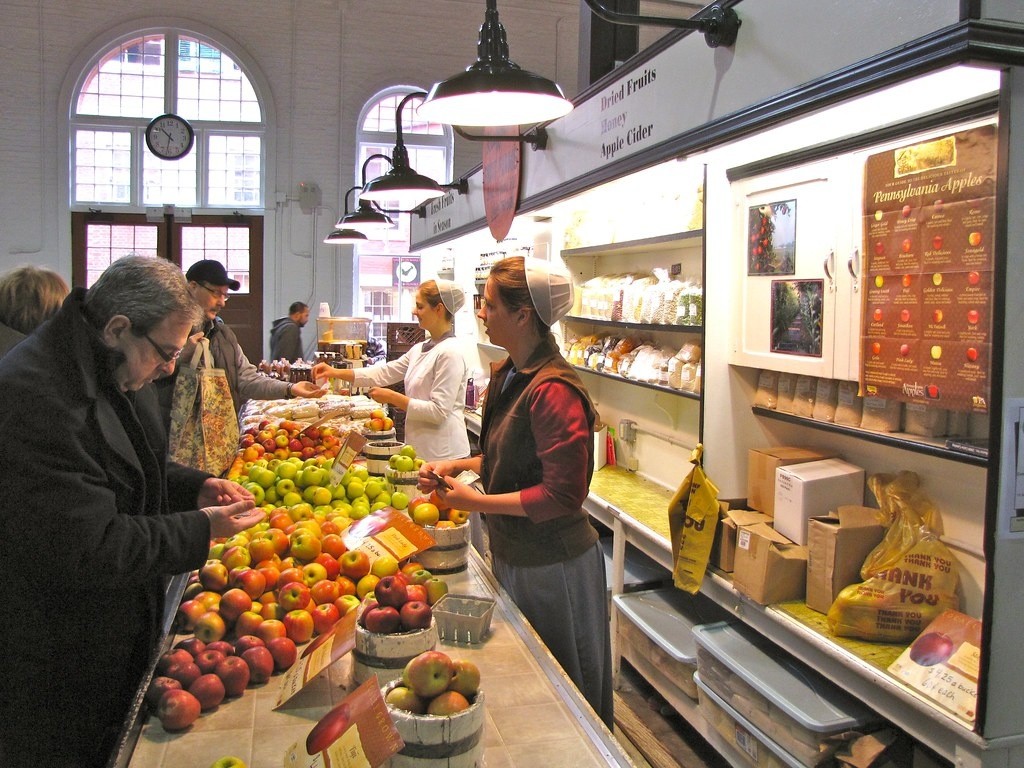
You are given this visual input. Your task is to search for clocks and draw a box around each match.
[146,114,194,160]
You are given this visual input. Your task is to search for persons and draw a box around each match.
[156,259,328,454]
[0,255,265,768]
[311,278,485,563]
[0,264,68,360]
[270,302,310,365]
[416,255,614,738]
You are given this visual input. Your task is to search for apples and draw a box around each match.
[148,408,481,731]
[210,754,245,767]
[751,218,768,270]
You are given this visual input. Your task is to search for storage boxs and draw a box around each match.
[613,586,885,768]
[387,323,425,443]
[594,424,607,471]
[709,447,887,614]
[430,593,496,643]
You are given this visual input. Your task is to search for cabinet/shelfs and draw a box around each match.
[475,279,507,352]
[560,228,702,399]
[728,109,999,384]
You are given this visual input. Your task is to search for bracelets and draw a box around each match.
[285,383,298,400]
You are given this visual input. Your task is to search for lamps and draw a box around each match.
[335,155,468,230]
[358,92,548,200]
[416,1,573,126]
[323,186,426,245]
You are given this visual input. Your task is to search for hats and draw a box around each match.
[436,277,466,314]
[186,259,240,291]
[524,256,575,327]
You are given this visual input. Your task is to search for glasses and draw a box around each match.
[139,329,180,367]
[193,279,230,301]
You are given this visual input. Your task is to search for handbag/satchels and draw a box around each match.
[166,338,239,478]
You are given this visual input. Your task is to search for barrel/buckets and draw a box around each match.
[386,464,427,500]
[410,519,471,576]
[361,427,397,457]
[366,440,405,477]
[351,614,438,688]
[379,677,485,768]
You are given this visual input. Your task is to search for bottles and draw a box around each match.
[466,378,475,407]
[317,354,347,390]
[257,357,315,383]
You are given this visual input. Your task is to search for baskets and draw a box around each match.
[432,593,496,645]
